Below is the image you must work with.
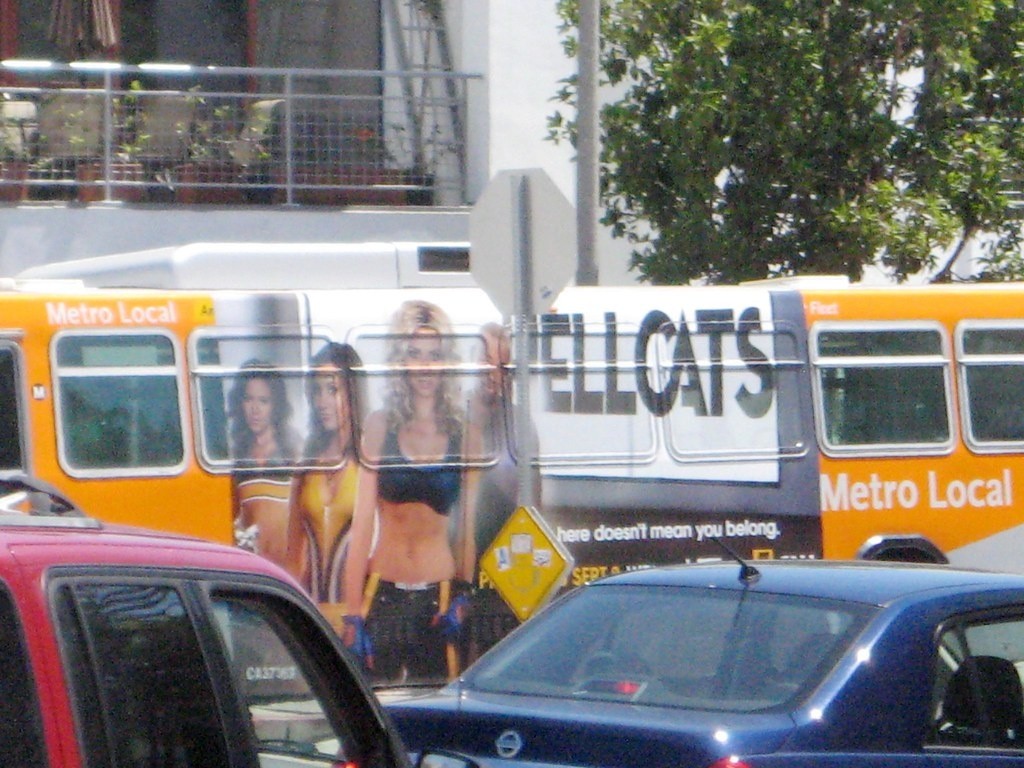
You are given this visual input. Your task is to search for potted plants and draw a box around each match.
[69,79,153,203]
[376,122,459,207]
[174,84,241,203]
[241,103,274,204]
[0,92,46,201]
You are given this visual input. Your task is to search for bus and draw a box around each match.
[0,238,518,738]
[535,276,1023,660]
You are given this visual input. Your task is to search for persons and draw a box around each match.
[224,297,544,689]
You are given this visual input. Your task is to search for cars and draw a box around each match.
[344,556,1024,768]
[0,470,414,768]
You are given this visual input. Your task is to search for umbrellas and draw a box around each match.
[47,0,122,55]
